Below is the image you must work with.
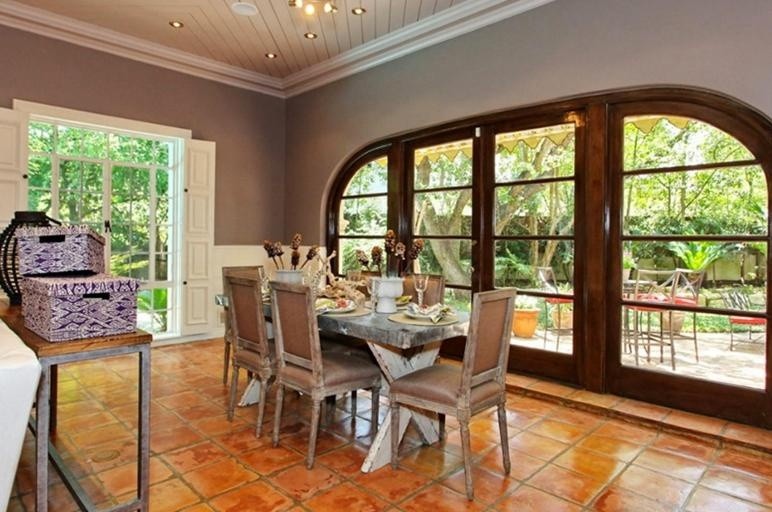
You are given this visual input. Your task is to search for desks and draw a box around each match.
[0,292,153,512]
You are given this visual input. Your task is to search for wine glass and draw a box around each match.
[411,274,430,308]
[365,280,381,316]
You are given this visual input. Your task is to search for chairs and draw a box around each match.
[350,274,445,437]
[221,266,270,394]
[268,280,381,470]
[388,287,516,501]
[222,274,327,439]
[538,268,768,375]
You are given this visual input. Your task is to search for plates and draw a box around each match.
[320,301,355,313]
[405,308,441,321]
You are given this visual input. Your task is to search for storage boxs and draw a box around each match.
[16,225,139,344]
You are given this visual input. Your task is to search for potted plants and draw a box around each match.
[512,301,540,339]
